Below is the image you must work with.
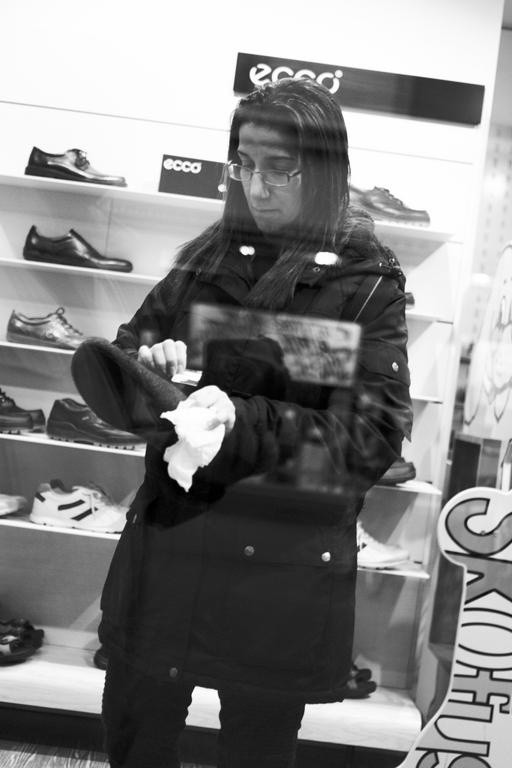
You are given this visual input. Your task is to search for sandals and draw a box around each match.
[0,614,45,668]
[342,659,377,698]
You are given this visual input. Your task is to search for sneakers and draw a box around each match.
[27,478,131,532]
[0,491,29,516]
[71,336,190,449]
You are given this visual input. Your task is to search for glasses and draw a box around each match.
[225,158,301,188]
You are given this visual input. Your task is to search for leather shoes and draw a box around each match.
[46,394,147,450]
[0,386,46,434]
[346,183,431,227]
[24,146,128,187]
[21,224,133,272]
[373,456,416,486]
[6,308,89,350]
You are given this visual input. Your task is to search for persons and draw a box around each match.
[93,76,414,767]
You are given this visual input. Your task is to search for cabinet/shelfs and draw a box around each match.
[0,97,471,766]
